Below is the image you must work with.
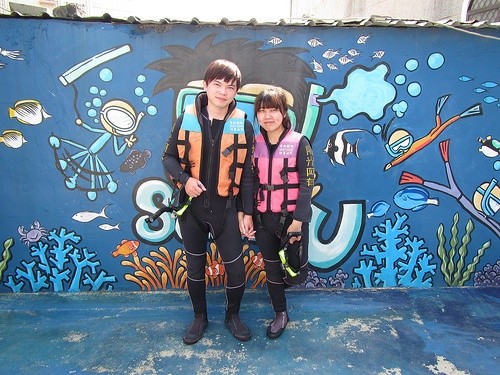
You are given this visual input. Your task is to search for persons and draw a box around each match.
[161,60,254,345]
[236,89,315,338]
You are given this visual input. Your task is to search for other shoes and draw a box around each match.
[266,311,290,339]
[182,313,208,343]
[224,313,252,341]
